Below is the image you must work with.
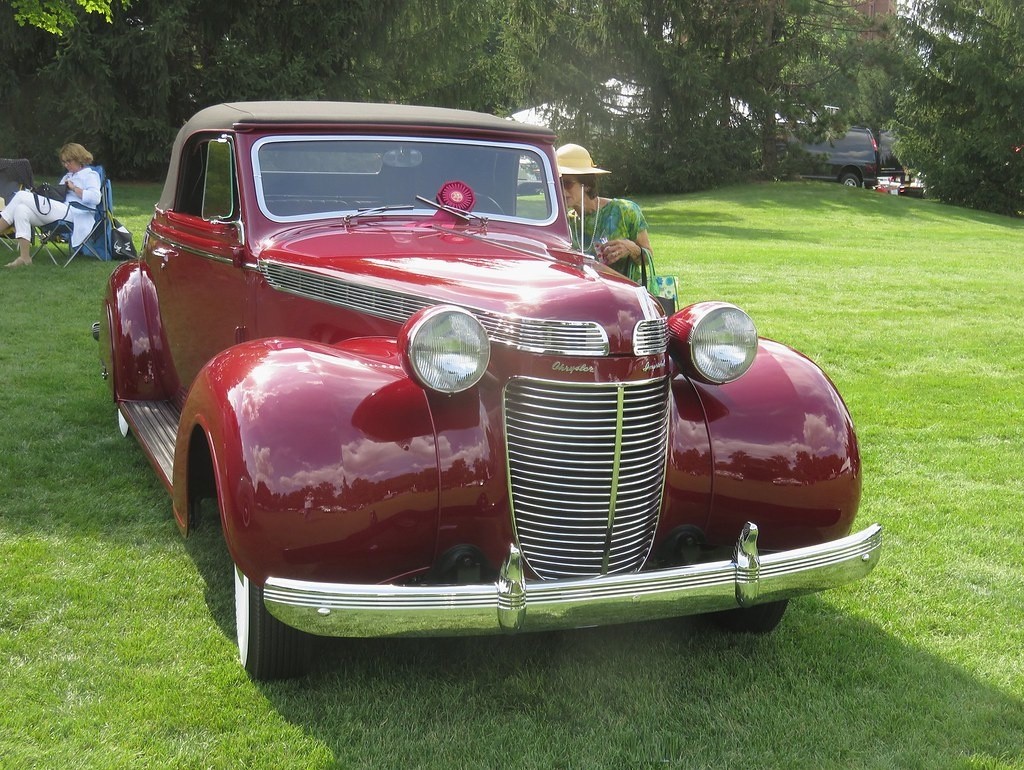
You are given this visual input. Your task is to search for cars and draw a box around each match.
[93,100,882,681]
[898,179,929,199]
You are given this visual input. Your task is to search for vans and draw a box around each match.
[775,122,879,189]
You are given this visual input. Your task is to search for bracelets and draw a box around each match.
[73,187,78,192]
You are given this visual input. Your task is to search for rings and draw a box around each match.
[615,251,618,256]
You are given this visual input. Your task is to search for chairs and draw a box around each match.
[0,157,34,251]
[266,160,483,216]
[31,165,113,269]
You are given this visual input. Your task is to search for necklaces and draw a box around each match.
[575,197,600,253]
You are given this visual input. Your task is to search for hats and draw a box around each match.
[533,144,612,174]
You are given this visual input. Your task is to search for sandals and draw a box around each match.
[4,257,33,267]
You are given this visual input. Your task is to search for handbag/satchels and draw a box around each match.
[33,182,67,215]
[624,246,678,317]
[107,210,137,260]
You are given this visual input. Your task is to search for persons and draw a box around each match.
[554,143,653,285]
[0,143,102,267]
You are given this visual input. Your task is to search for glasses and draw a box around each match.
[61,158,74,165]
[560,180,578,190]
